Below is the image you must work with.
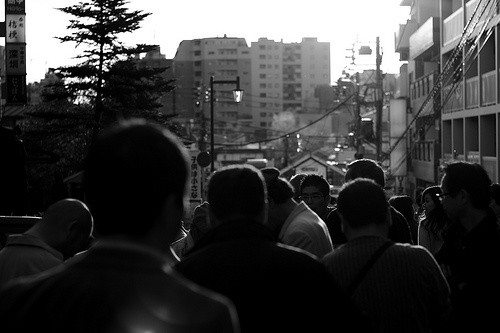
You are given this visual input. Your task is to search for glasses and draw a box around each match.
[436,189,465,202]
[299,195,324,201]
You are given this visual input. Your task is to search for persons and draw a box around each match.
[0,117,240,332]
[175,159,500,332]
[1,198,95,285]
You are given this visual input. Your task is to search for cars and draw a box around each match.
[0,215,41,248]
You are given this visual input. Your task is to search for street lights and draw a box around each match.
[209,74,245,171]
